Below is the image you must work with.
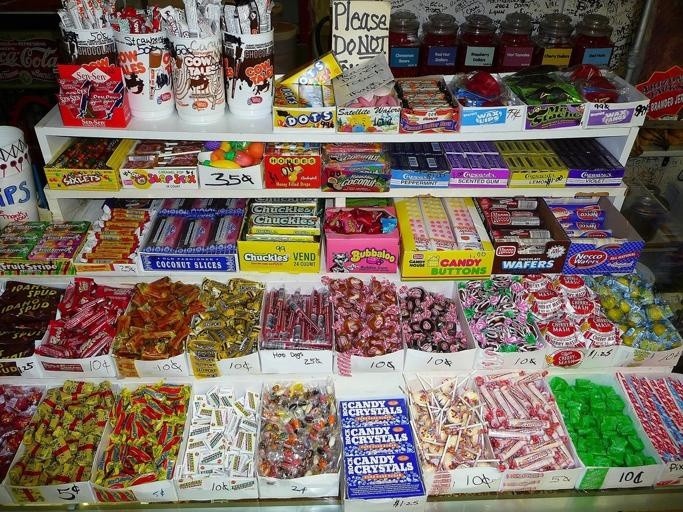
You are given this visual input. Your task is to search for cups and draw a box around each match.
[0,123,40,225]
[60,17,280,125]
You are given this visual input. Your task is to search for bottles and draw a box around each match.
[389,11,614,69]
[624,185,670,242]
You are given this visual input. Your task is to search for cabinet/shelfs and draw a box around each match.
[0,1,682,510]
